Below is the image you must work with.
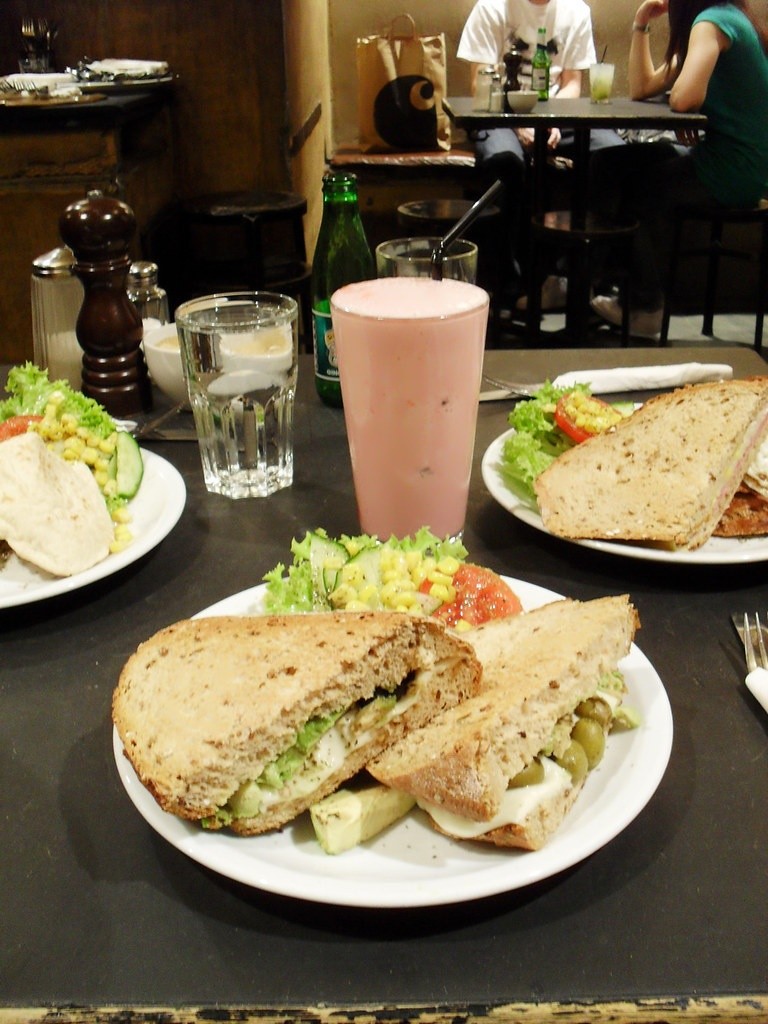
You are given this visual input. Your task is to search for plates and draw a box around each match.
[0,404,187,612]
[111,551,673,907]
[69,68,175,89]
[482,390,768,569]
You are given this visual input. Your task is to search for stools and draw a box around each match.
[184,190,311,347]
[540,209,642,336]
[658,198,768,343]
[399,200,502,243]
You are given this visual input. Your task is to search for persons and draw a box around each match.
[456,0,628,277]
[589,0,768,338]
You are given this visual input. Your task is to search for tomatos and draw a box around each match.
[412,563,522,624]
[0,414,44,442]
[553,394,622,442]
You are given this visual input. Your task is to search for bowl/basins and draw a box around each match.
[373,235,478,299]
[507,89,539,114]
[142,320,291,404]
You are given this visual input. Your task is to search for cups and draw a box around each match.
[175,289,302,497]
[588,64,615,104]
[329,276,489,565]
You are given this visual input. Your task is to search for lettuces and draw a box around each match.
[261,528,467,615]
[0,360,120,441]
[499,377,633,492]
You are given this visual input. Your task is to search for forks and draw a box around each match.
[0,79,51,99]
[743,611,768,680]
[484,373,538,398]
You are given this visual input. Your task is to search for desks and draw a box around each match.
[442,96,709,345]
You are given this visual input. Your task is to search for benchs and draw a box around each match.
[332,144,503,223]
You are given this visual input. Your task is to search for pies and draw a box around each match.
[1,432,116,579]
[710,480,767,536]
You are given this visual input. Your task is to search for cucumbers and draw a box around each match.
[113,430,143,498]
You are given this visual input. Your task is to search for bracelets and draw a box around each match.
[633,24,650,32]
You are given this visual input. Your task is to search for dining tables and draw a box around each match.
[1,347,768,1024]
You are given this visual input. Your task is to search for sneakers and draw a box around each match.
[591,295,663,334]
[515,275,568,310]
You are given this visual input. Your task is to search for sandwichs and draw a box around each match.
[366,594,639,851]
[110,611,482,835]
[533,374,768,550]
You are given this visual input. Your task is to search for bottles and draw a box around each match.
[474,66,505,114]
[310,171,387,411]
[122,260,171,335]
[30,246,87,394]
[531,27,550,101]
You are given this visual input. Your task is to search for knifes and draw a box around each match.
[477,365,736,405]
[731,611,768,669]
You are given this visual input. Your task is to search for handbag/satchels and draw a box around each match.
[355,14,452,153]
